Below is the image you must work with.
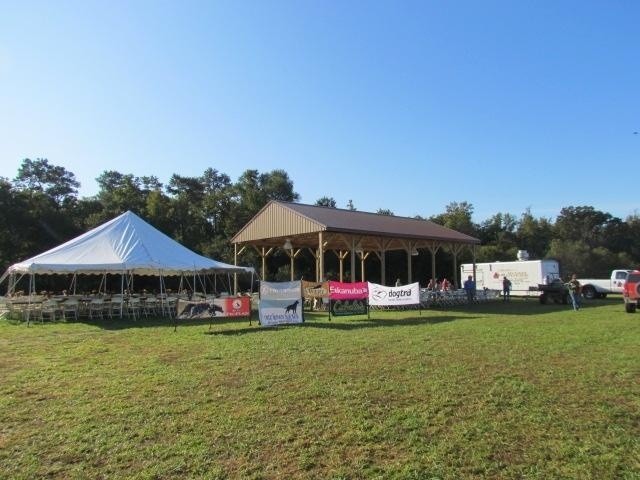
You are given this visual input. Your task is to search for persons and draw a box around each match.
[502,276,510,303]
[428,278,454,299]
[464,276,476,303]
[395,278,401,287]
[568,274,581,311]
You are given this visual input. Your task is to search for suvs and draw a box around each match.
[624,272,640,313]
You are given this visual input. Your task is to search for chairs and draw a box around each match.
[420,290,497,308]
[7,291,175,324]
[250,291,259,313]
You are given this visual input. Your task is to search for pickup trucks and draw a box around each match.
[573,268,638,301]
